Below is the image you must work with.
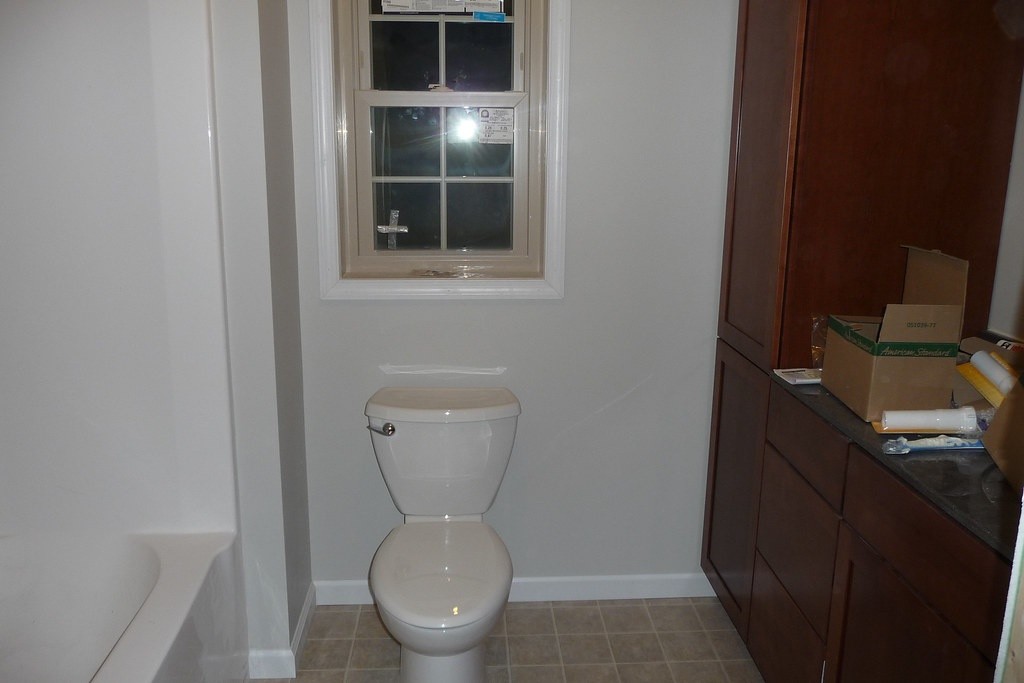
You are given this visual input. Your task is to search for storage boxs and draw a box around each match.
[819,245,969,423]
[981,375,1024,493]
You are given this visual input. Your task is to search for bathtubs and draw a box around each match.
[1,531,243,683]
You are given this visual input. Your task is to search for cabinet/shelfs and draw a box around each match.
[698,338,771,647]
[745,378,853,683]
[820,443,1012,683]
[716,0,1024,380]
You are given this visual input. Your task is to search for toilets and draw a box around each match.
[363,366,522,681]
